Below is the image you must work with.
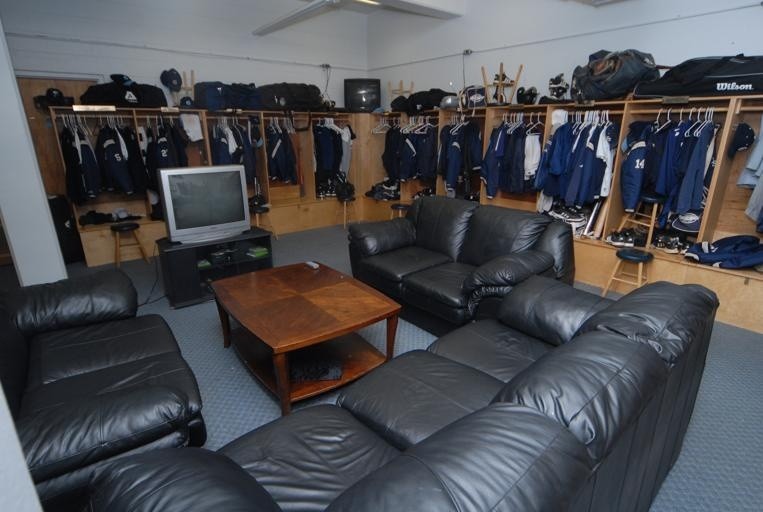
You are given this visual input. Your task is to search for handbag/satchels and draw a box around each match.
[575,48,761,101]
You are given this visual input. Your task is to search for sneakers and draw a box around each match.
[546,201,588,228]
[367,176,400,201]
[515,72,572,107]
[317,172,348,199]
[602,226,690,257]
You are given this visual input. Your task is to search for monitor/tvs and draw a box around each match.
[344,78,381,112]
[155,163,251,245]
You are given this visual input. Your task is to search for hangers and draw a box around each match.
[490,109,616,138]
[61,113,345,141]
[638,106,720,138]
[369,115,483,138]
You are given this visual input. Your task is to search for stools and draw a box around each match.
[110,223,151,270]
[389,204,411,220]
[170,70,195,109]
[617,190,667,248]
[481,62,524,106]
[601,248,653,298]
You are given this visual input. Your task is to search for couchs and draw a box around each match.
[83,272,720,511]
[347,196,574,337]
[0,266,204,474]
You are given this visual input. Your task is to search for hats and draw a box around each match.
[726,122,755,158]
[669,212,704,232]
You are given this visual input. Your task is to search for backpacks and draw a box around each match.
[40,69,331,114]
[389,84,459,110]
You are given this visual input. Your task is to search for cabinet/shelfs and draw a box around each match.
[365,95,762,333]
[155,225,274,309]
[49,106,364,267]
